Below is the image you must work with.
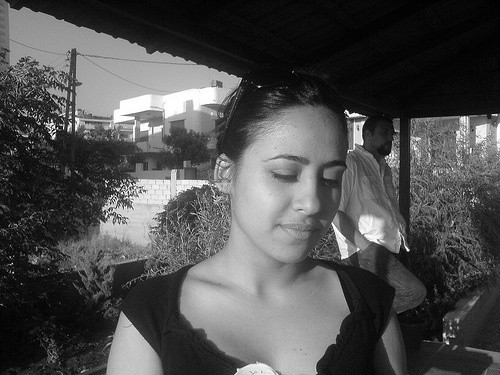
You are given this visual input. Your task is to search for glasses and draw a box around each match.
[218,69,339,148]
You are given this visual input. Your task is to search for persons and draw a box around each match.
[105,68,406,375]
[330,114,427,316]
